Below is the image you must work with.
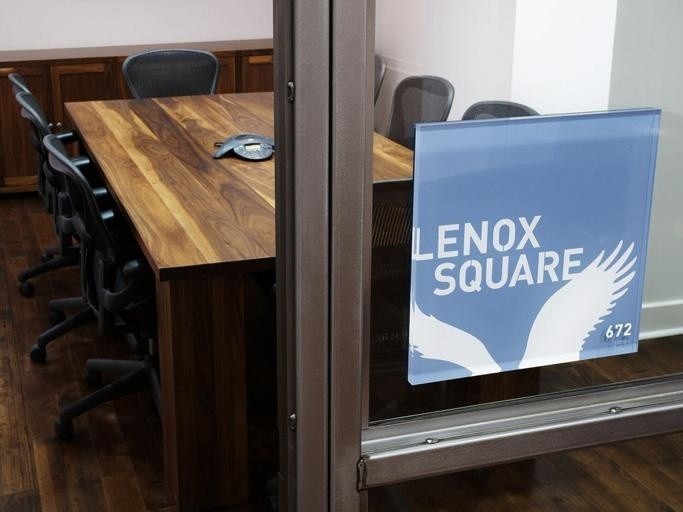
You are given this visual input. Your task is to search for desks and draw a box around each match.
[62,92,415,511]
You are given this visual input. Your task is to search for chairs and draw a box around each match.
[0,39,157,439]
[461,100,541,120]
[384,75,455,151]
[366,179,413,422]
[374,54,387,107]
[122,50,220,99]
